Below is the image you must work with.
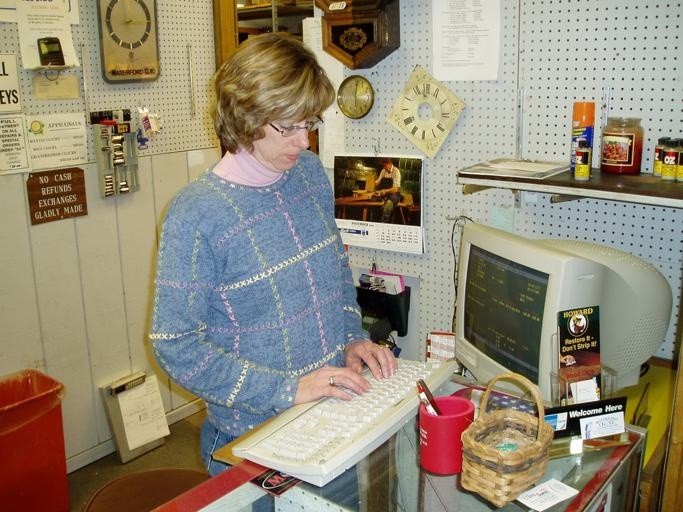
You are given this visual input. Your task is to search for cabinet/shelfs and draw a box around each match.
[154,376,647,512]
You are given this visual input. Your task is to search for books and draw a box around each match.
[460,152,571,181]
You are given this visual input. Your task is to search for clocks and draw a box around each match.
[337,75,374,118]
[96,1,161,85]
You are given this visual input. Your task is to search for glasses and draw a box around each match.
[269,113,325,137]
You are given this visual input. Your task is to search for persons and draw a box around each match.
[149,30,397,512]
[369,157,401,223]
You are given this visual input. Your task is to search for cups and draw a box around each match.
[417,395,475,475]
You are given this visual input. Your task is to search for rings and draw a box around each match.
[379,342,391,351]
[330,377,335,386]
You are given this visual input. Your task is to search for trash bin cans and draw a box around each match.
[0,368,70,512]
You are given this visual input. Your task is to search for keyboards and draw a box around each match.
[231,358,460,488]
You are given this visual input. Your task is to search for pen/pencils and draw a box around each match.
[418,379,444,416]
[416,382,439,416]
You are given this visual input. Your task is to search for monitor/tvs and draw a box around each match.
[454,223,674,406]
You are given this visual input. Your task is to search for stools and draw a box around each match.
[84,469,202,512]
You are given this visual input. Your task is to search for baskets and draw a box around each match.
[460,375,553,509]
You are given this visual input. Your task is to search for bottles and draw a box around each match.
[574,137,592,183]
[599,116,644,172]
[652,137,683,183]
